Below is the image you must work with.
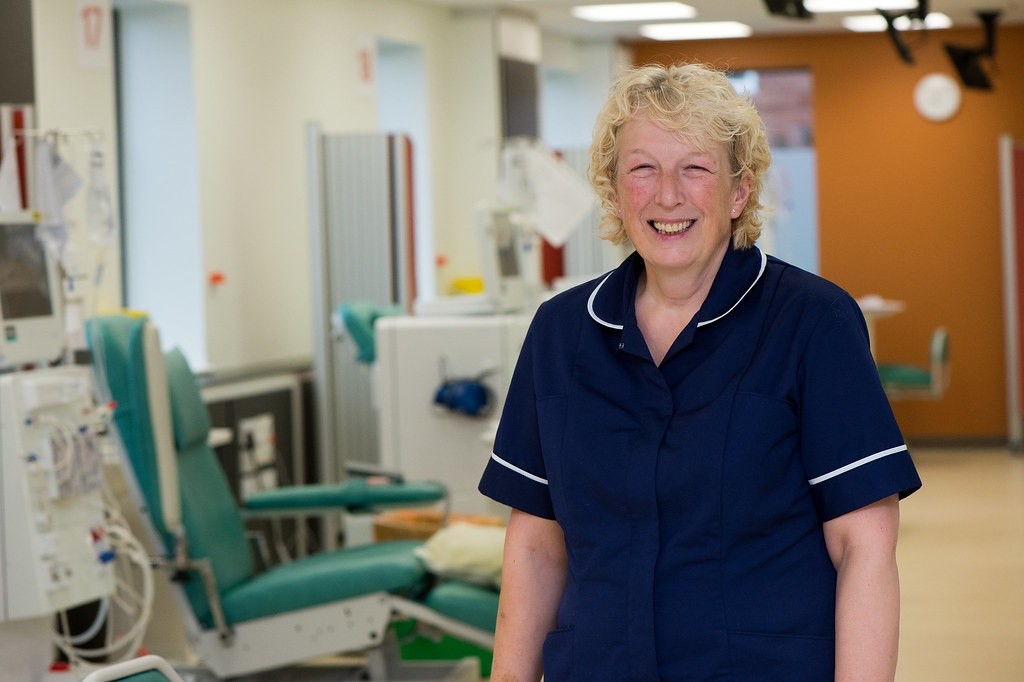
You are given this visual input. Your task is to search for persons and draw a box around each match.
[477,64,922,682]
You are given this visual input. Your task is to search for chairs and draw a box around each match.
[873,325,950,411]
[85,305,501,682]
[333,298,408,367]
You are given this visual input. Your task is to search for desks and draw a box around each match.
[372,313,537,525]
